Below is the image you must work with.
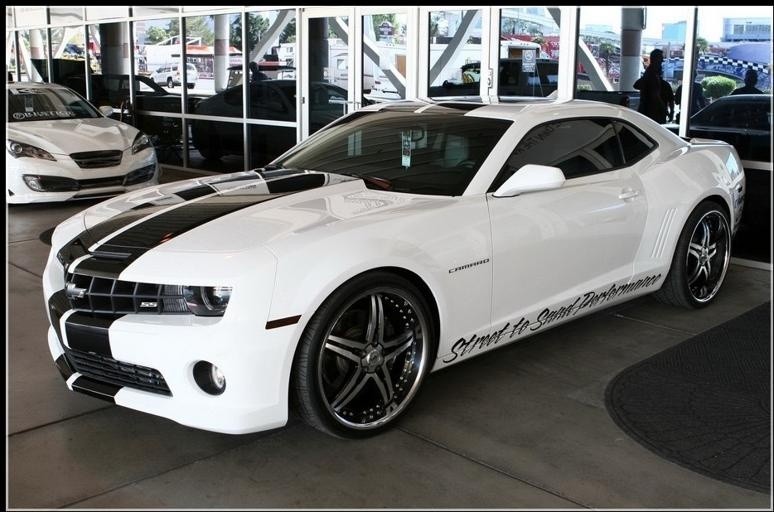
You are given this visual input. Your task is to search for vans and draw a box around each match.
[150,62,198,89]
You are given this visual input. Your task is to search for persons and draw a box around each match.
[248,60,269,82]
[675,69,707,113]
[634,65,673,124]
[643,49,665,81]
[730,69,764,95]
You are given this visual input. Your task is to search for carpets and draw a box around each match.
[40,227,55,245]
[606,299,770,496]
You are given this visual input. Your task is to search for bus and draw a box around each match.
[277,42,373,94]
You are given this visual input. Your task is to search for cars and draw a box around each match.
[429,61,630,109]
[42,101,746,439]
[191,78,373,163]
[44,74,207,158]
[659,94,772,160]
[6,81,158,205]
